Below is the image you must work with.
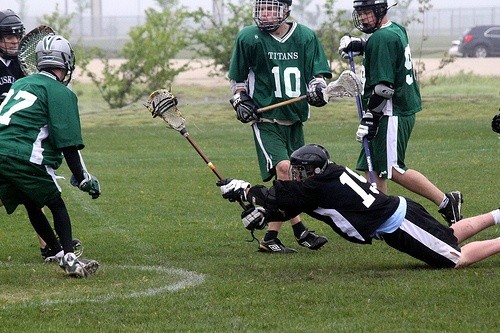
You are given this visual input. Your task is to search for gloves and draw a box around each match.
[340,33,363,58]
[356,109,385,143]
[216,177,250,203]
[71,170,101,199]
[241,205,268,232]
[306,74,330,107]
[229,91,262,124]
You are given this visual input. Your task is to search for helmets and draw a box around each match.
[36,33,75,69]
[0,8,24,34]
[353,0,387,8]
[256,0,292,6]
[290,144,329,175]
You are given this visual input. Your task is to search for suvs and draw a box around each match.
[457,24,500,58]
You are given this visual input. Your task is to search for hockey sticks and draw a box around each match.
[256,70,364,113]
[17,25,96,197]
[349,48,378,188]
[147,89,248,212]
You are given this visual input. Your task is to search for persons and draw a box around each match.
[228,0,332,253]
[216,144,500,267]
[0,34,100,277]
[0,10,83,262]
[338,0,464,227]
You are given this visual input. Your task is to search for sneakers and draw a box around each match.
[257,237,297,254]
[296,228,328,251]
[437,191,464,226]
[39,228,81,262]
[59,249,100,279]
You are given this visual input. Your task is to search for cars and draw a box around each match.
[449,39,471,57]
